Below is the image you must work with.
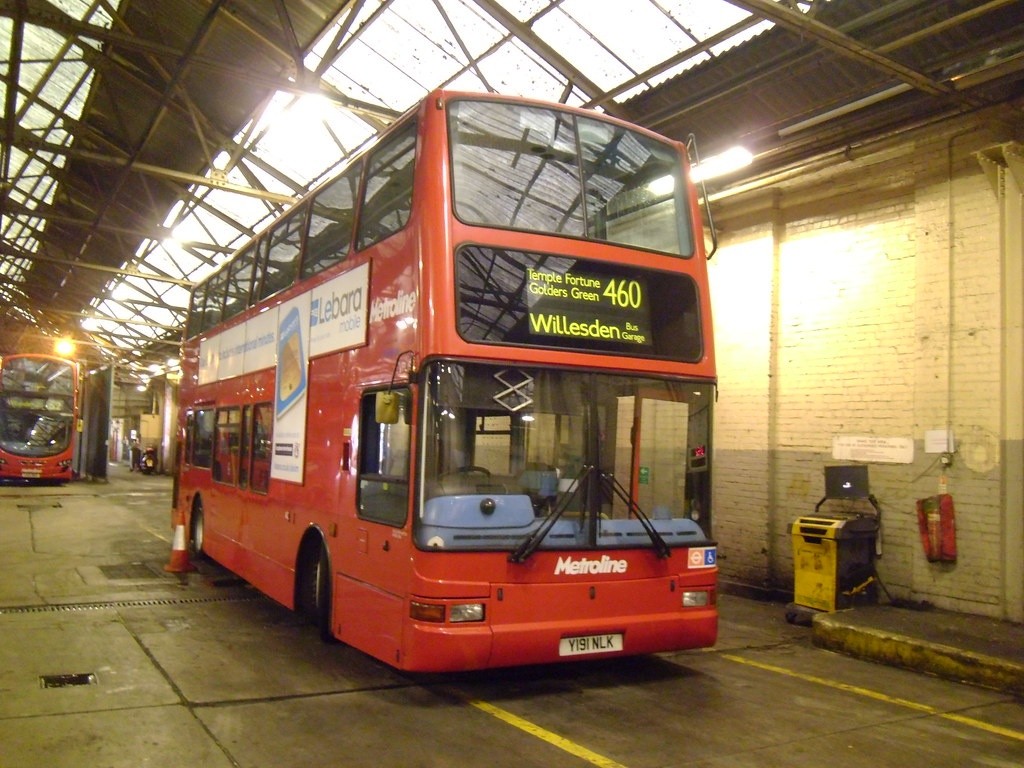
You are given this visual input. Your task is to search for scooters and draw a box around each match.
[139,443,159,474]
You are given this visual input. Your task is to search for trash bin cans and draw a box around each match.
[790,512,881,613]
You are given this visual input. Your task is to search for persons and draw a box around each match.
[124,434,142,472]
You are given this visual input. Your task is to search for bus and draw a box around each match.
[169,101,721,678]
[0,354,81,485]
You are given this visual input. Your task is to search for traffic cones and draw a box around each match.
[165,505,195,574]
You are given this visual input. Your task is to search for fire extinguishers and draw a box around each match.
[925,496,943,563]
